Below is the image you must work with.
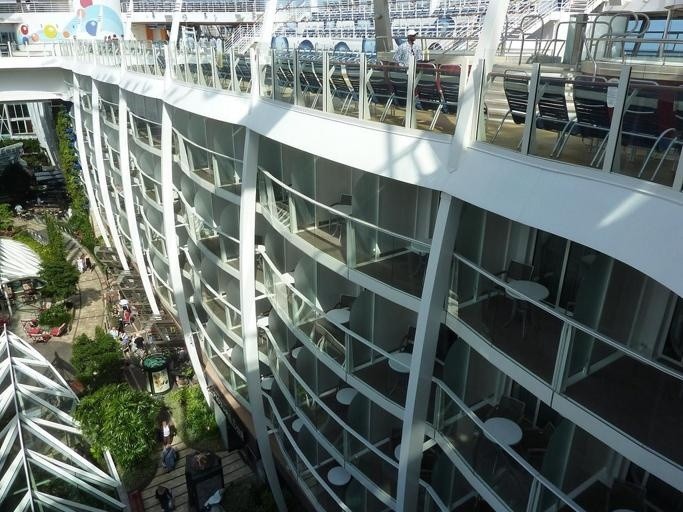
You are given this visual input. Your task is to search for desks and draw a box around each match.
[256,201,550,487]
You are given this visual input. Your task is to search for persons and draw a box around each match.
[14,204,22,215]
[77,257,84,273]
[155,485,174,512]
[109,306,145,352]
[104,34,124,47]
[160,443,177,473]
[187,34,217,49]
[393,30,422,67]
[160,421,173,446]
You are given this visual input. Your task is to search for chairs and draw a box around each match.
[157,51,683,181]
[602,475,647,511]
